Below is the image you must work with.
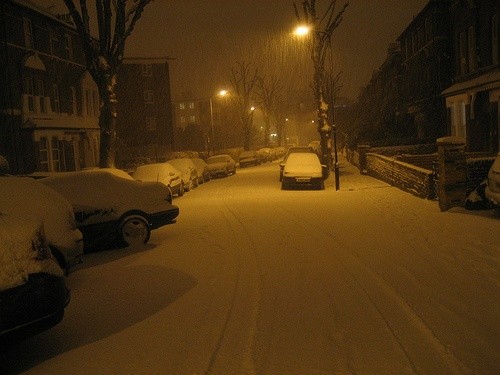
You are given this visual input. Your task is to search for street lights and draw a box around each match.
[295,26,340,191]
[209,90,226,155]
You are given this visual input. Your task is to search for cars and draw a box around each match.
[0,176,84,340]
[237,147,326,179]
[206,155,237,178]
[131,164,186,198]
[279,152,326,190]
[35,169,180,249]
[167,158,212,191]
